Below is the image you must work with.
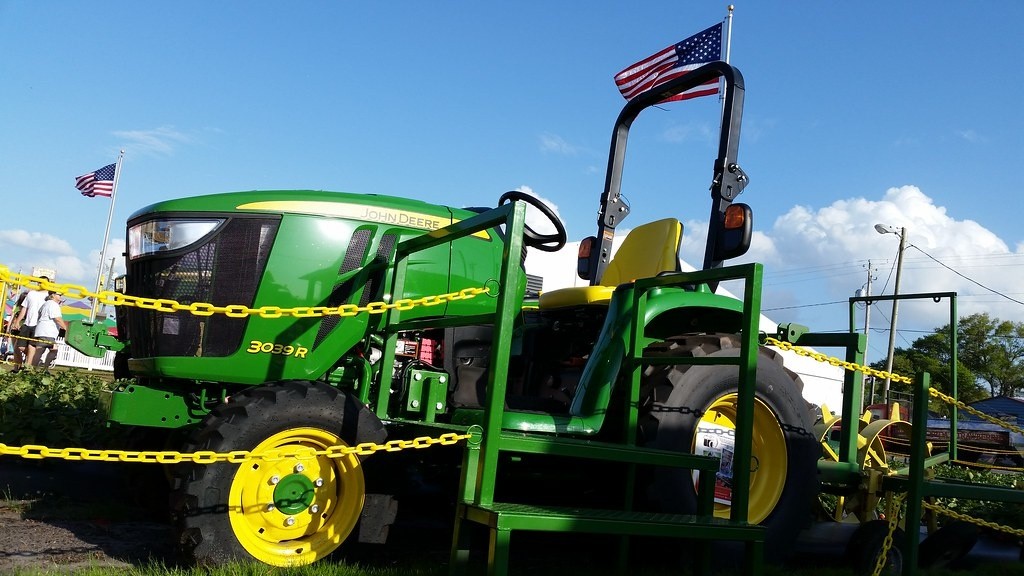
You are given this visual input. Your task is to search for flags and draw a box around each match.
[613,21,724,106]
[74,162,116,199]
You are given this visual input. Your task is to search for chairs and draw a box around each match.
[539,216,686,352]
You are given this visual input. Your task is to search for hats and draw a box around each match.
[49,291,63,295]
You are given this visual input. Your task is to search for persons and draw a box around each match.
[8,275,67,374]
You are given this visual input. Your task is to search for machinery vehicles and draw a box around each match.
[63,63,959,576]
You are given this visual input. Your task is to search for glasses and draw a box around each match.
[56,294,62,296]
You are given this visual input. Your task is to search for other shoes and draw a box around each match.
[20,367,25,370]
[10,370,17,374]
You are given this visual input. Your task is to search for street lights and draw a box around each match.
[875,222,909,406]
